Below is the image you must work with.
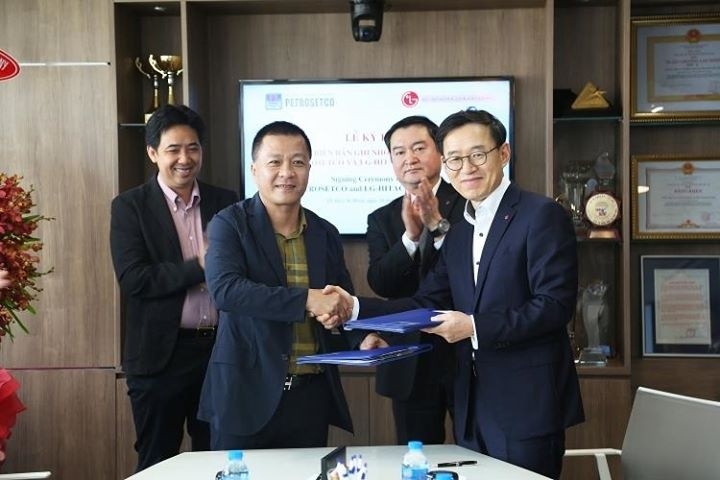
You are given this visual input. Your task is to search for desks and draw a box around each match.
[123,444,554,480]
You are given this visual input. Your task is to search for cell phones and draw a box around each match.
[428,471,459,480]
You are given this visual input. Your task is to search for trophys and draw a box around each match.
[556,151,620,239]
[136,52,183,125]
[567,279,610,367]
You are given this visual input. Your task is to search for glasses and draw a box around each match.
[443,142,504,171]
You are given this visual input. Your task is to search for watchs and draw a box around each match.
[428,218,450,237]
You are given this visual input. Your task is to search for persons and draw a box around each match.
[305,106,588,480]
[110,106,242,477]
[363,117,463,447]
[198,120,389,449]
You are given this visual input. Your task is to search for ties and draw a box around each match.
[418,226,428,260]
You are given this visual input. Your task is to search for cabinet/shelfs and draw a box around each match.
[111,1,719,380]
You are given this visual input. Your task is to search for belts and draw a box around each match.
[179,326,217,339]
[283,373,327,391]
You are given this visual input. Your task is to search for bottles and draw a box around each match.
[221,451,250,480]
[401,441,429,480]
[436,473,453,480]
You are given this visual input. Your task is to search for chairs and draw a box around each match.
[564,386,720,480]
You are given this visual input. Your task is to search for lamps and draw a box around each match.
[350,0,384,42]
